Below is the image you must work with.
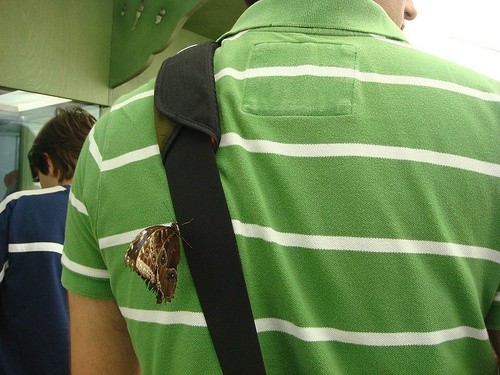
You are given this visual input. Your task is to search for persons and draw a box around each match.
[60,0,500,375]
[0,107,98,375]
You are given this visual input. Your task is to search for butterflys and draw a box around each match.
[123,201,194,305]
